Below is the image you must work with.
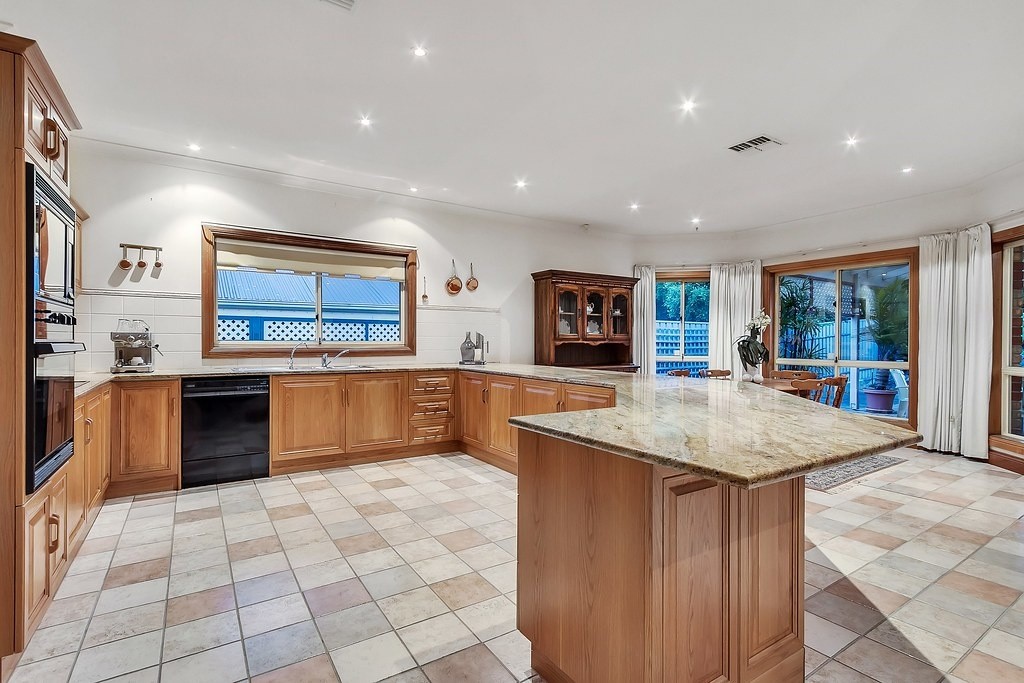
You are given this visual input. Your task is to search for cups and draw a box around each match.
[615,309,620,313]
[587,302,594,314]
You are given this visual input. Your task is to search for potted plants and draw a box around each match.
[860,274,910,412]
[734,306,773,364]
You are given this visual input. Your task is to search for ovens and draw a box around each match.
[25,339,88,498]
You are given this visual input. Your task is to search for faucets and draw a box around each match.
[319,349,351,368]
[287,341,309,370]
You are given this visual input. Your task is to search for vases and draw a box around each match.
[740,355,764,383]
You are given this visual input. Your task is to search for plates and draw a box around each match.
[559,318,570,334]
[613,312,622,315]
[586,319,600,334]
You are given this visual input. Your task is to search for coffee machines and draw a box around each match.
[111,318,158,372]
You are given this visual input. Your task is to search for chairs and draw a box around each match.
[771,367,848,409]
[668,368,734,383]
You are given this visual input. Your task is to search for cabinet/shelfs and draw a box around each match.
[0,29,918,683]
[531,268,641,375]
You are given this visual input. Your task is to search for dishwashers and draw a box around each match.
[180,375,270,490]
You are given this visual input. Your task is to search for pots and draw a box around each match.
[446,259,462,295]
[465,263,478,290]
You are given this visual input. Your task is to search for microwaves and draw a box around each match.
[25,164,77,341]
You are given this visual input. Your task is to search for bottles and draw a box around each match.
[130,356,143,366]
[460,332,475,362]
